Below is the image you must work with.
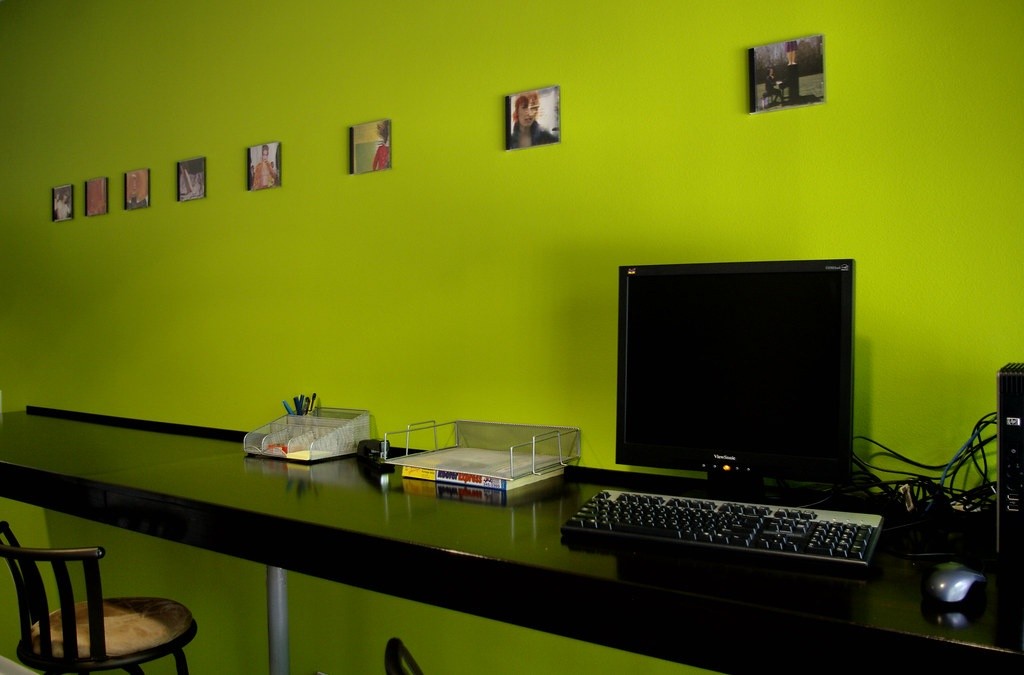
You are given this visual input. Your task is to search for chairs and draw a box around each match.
[0,520,197,675]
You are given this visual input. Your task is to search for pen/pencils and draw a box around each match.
[283,400,295,414]
[294,392,317,416]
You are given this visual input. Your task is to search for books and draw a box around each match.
[402,446,564,490]
[402,475,560,507]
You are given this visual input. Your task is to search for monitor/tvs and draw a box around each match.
[617,259,854,505]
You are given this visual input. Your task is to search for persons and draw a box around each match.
[54,192,71,219]
[510,92,557,148]
[766,68,786,104]
[785,40,798,65]
[252,144,277,189]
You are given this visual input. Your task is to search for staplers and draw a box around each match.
[357,439,390,463]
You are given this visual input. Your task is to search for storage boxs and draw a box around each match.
[240,405,370,463]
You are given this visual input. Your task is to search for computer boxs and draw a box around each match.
[996,363,1023,557]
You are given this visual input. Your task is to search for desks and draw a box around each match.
[0,413,1024,675]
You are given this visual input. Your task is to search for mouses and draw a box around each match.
[921,560,990,630]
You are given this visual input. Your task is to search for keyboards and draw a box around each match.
[561,491,886,577]
